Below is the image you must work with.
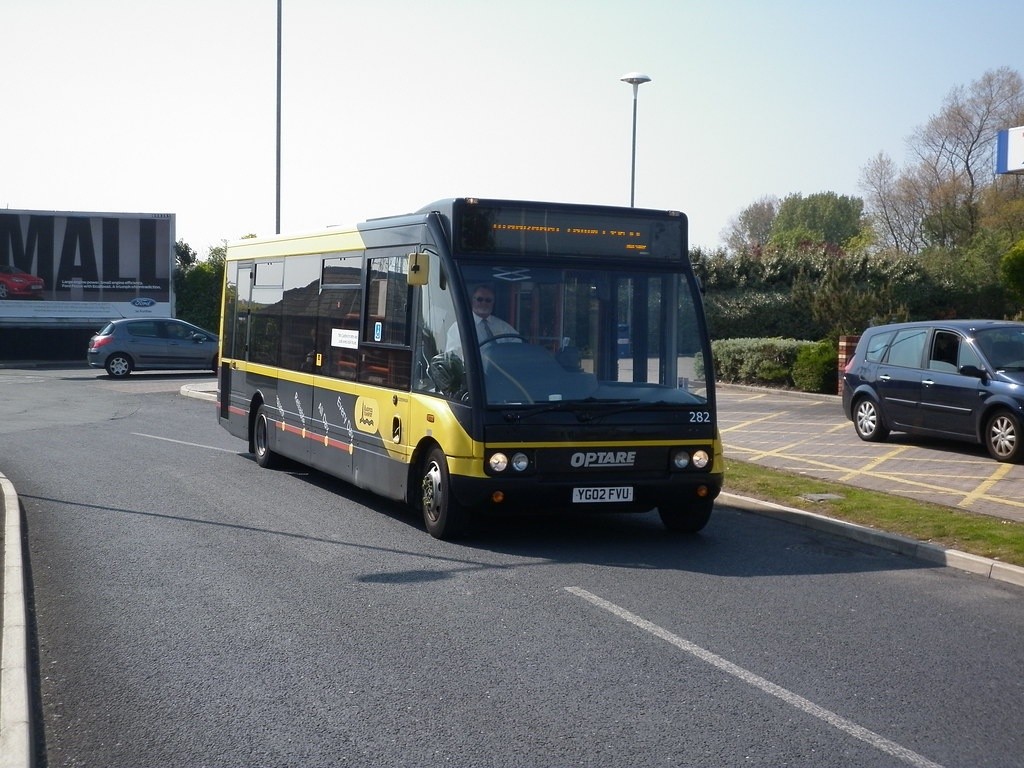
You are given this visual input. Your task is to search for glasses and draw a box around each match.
[473,296,494,303]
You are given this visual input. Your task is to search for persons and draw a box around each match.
[166,322,184,338]
[301,327,330,375]
[445,285,523,376]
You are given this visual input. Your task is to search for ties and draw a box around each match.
[482,318,497,345]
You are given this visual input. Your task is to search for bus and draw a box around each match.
[216,196,727,539]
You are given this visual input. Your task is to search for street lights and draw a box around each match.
[619,72,651,210]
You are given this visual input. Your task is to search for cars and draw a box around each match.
[86,318,219,379]
[0,265,44,298]
[841,319,1024,463]
